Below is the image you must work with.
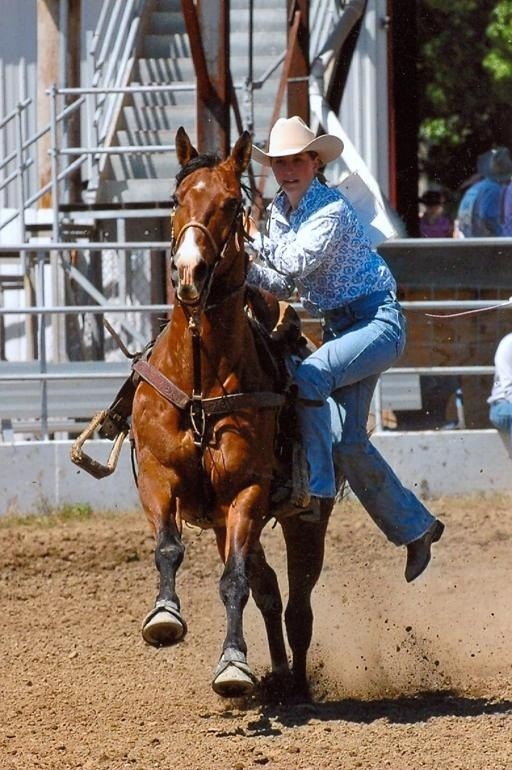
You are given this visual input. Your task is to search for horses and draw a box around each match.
[128,126,374,712]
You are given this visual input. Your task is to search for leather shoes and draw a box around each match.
[403,518,444,584]
[268,487,322,523]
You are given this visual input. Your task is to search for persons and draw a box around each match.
[417,192,454,239]
[239,115,444,583]
[487,333,512,429]
[454,145,512,239]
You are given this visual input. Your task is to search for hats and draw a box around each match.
[477,146,512,184]
[416,189,453,206]
[250,116,346,168]
